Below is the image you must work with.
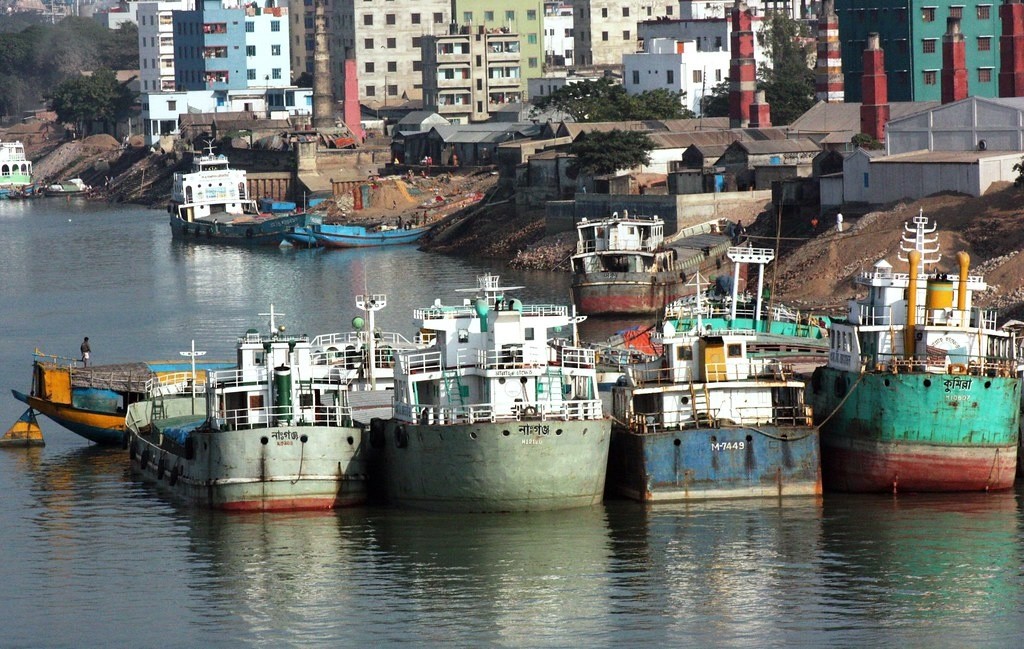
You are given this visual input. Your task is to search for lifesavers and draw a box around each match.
[946,363,969,376]
[524,406,538,420]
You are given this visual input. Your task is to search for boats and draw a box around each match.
[303,295,416,421]
[369,276,614,513]
[454,271,526,292]
[997,319,1023,374]
[567,212,752,314]
[9,350,239,449]
[660,285,830,351]
[805,209,1021,493]
[613,270,824,501]
[0,140,37,199]
[44,179,89,196]
[285,223,427,248]
[168,141,323,247]
[5,192,42,199]
[129,306,363,513]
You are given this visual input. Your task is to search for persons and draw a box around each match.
[10,183,42,196]
[424,210,428,226]
[811,216,818,237]
[732,220,745,244]
[81,337,91,368]
[407,169,413,179]
[393,200,396,210]
[818,317,827,329]
[397,216,402,229]
[160,147,165,154]
[404,220,415,230]
[836,211,843,232]
[416,212,420,226]
